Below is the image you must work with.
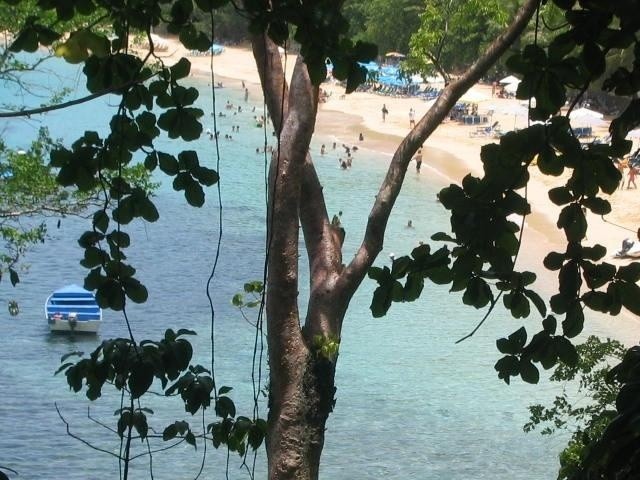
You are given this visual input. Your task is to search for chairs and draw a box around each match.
[373,85,591,139]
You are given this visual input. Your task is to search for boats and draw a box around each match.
[45,284,102,334]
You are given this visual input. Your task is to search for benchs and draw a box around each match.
[47,297,99,316]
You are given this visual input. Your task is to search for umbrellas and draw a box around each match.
[500,75,519,84]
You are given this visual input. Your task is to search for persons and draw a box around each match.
[199,66,423,177]
[404,221,415,228]
[388,252,395,261]
[617,164,624,189]
[626,166,638,188]
[337,212,342,223]
